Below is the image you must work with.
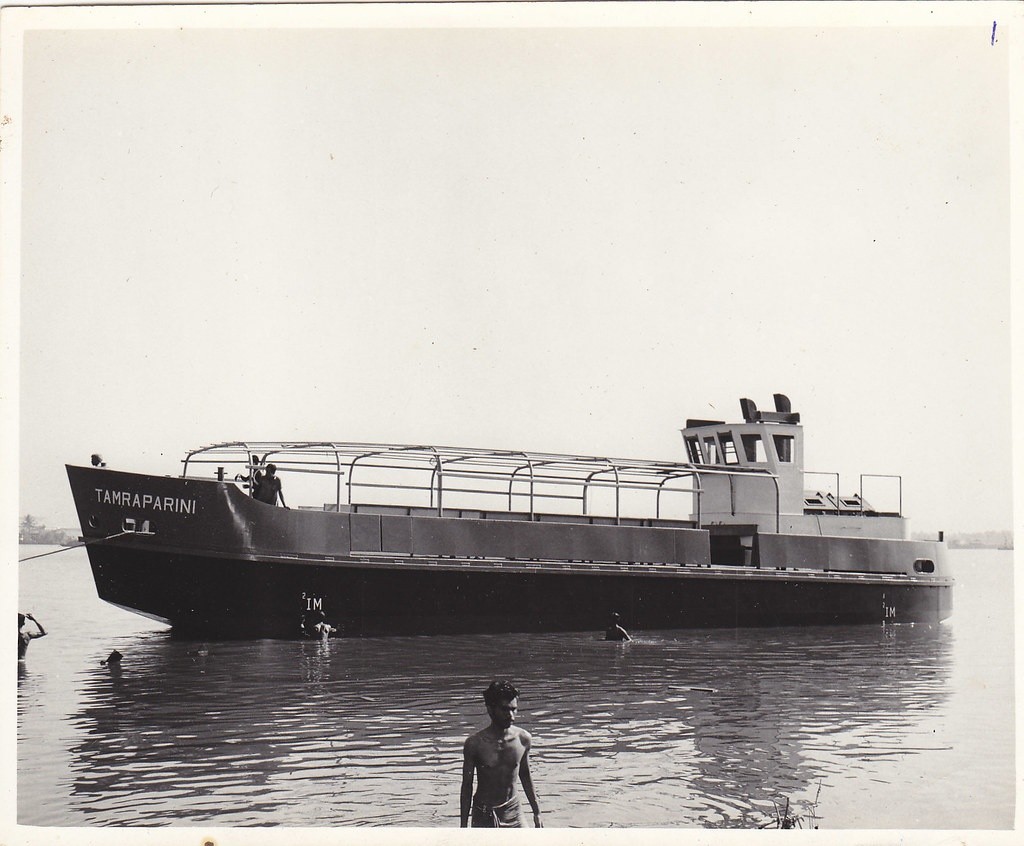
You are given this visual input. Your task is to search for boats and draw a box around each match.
[62,394,956,638]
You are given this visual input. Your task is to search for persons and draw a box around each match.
[605,608,632,641]
[18,612,48,660]
[300,609,340,641]
[460,680,544,828]
[235,455,290,510]
